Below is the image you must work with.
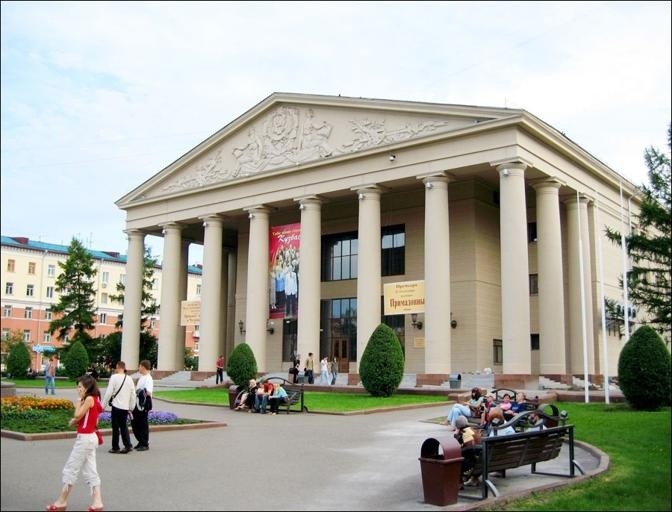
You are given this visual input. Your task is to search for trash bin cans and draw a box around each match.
[418,436,464,507]
[289,368,298,383]
[449,374,461,389]
[229,385,242,409]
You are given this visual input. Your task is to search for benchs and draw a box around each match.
[486,388,539,433]
[231,376,306,415]
[457,422,577,501]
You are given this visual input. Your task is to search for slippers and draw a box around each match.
[87,506,101,511]
[46,504,65,511]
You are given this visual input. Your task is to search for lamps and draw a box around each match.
[267,319,274,334]
[410,314,423,329]
[450,311,457,329]
[238,319,246,333]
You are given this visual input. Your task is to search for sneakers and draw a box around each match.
[108,444,149,454]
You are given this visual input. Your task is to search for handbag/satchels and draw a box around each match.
[109,395,115,406]
[95,431,103,445]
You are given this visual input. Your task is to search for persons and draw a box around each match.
[331,356,338,385]
[304,352,314,384]
[130,360,153,451]
[275,244,299,316]
[319,356,330,385]
[293,354,301,383]
[215,355,225,384]
[438,388,548,487]
[234,379,289,415]
[43,360,56,395]
[45,374,104,512]
[102,361,137,454]
